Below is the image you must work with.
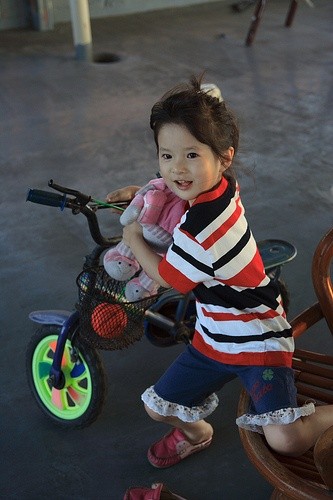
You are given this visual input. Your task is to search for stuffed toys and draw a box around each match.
[103,178,189,309]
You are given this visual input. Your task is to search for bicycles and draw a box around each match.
[25,179,299,433]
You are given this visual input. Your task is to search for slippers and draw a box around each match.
[122,482,187,500]
[146,427,212,469]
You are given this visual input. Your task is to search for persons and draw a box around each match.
[107,70,333,468]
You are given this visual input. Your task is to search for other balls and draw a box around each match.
[91,301,129,338]
